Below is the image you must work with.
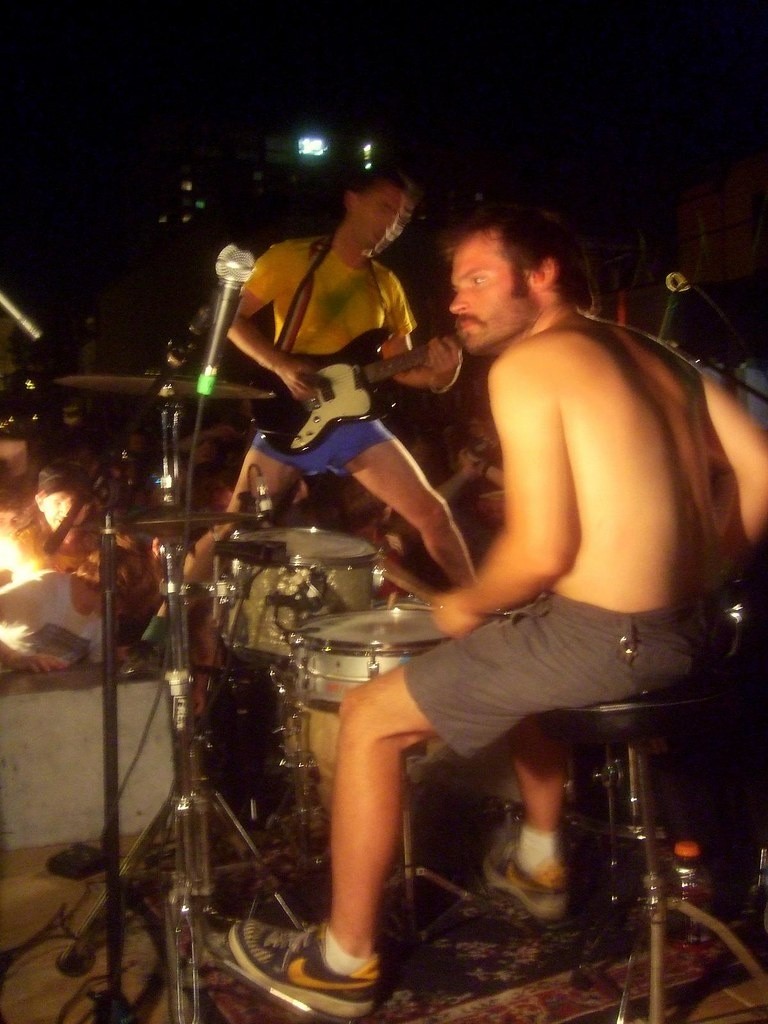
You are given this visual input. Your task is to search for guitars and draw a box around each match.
[242,325,463,456]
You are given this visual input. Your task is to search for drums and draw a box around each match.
[213,528,375,662]
[267,604,484,821]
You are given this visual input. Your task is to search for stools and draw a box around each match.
[537,697,768,1024]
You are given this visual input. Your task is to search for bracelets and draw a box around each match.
[429,382,448,394]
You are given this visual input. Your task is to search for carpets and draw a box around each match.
[130,831,768,1024]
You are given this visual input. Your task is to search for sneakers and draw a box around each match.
[481,828,569,921]
[228,918,380,1019]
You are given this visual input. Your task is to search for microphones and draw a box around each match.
[194,242,257,394]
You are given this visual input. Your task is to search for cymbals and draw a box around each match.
[52,370,275,403]
[99,510,261,538]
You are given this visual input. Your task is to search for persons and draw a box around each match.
[0,206,768,1018]
[141,166,478,644]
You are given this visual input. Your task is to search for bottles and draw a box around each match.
[751,849,768,941]
[670,840,710,902]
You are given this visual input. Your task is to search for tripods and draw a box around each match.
[56,382,593,1024]
